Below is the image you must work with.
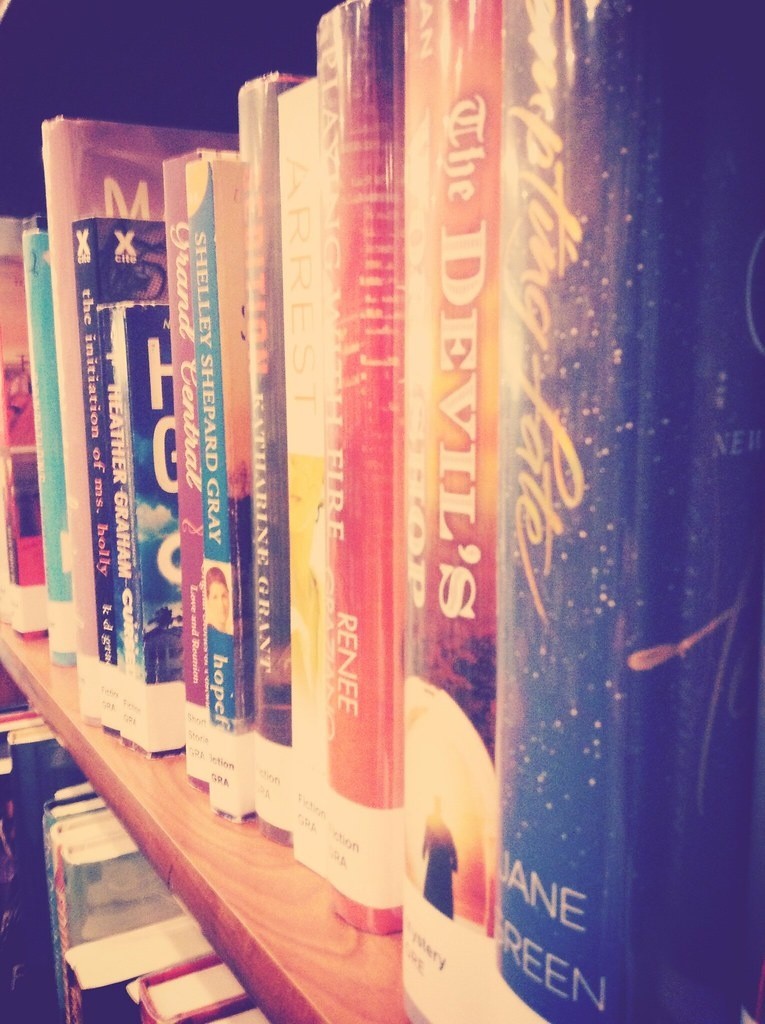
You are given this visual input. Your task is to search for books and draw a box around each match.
[0,0,765,1024]
